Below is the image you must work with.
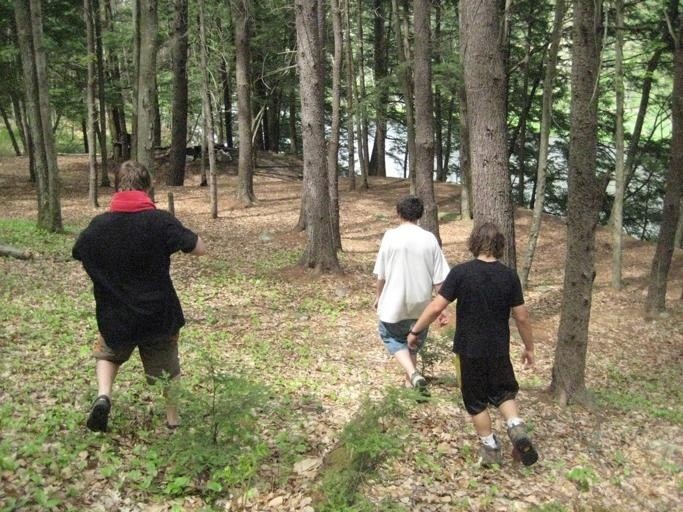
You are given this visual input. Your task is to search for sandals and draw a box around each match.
[87,395,111,432]
[164,420,179,435]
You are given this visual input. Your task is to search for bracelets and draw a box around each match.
[409,330,423,335]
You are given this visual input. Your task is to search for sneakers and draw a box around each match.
[474,434,502,460]
[506,422,539,467]
[410,372,430,404]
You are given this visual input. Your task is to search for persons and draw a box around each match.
[70,160,205,429]
[406,222,539,466]
[375,196,450,390]
